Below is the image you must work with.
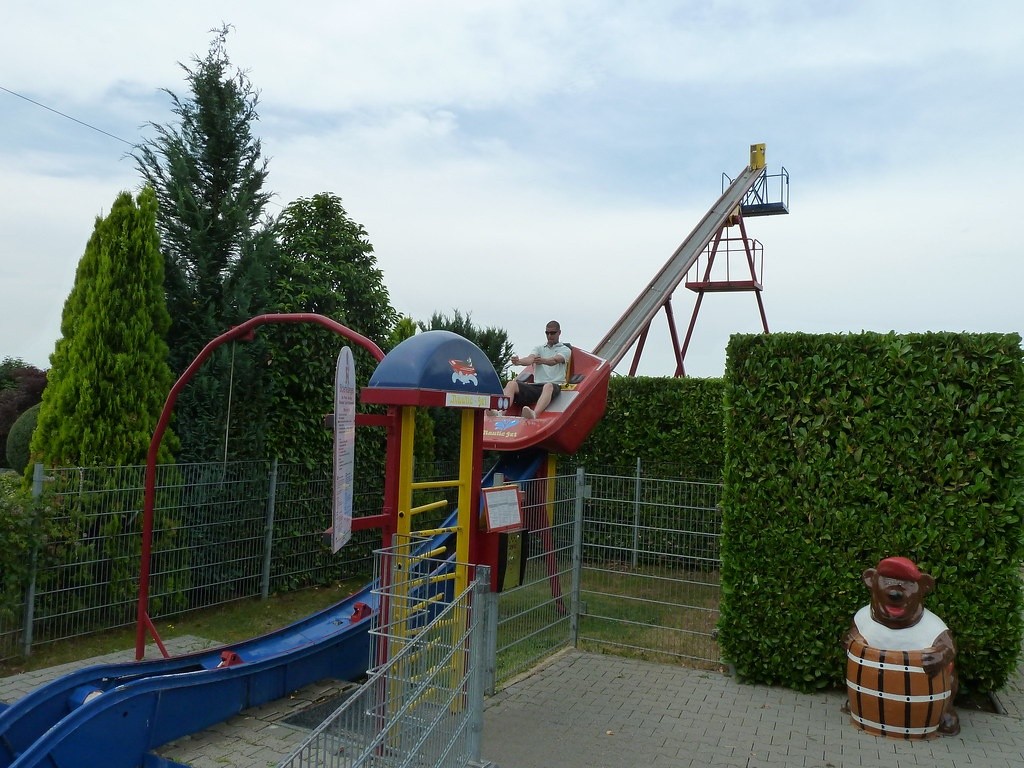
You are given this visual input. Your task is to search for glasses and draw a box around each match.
[545,331,558,335]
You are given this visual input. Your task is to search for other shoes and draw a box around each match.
[522,406,537,419]
[486,408,503,417]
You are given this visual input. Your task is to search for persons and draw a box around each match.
[488,321,571,419]
